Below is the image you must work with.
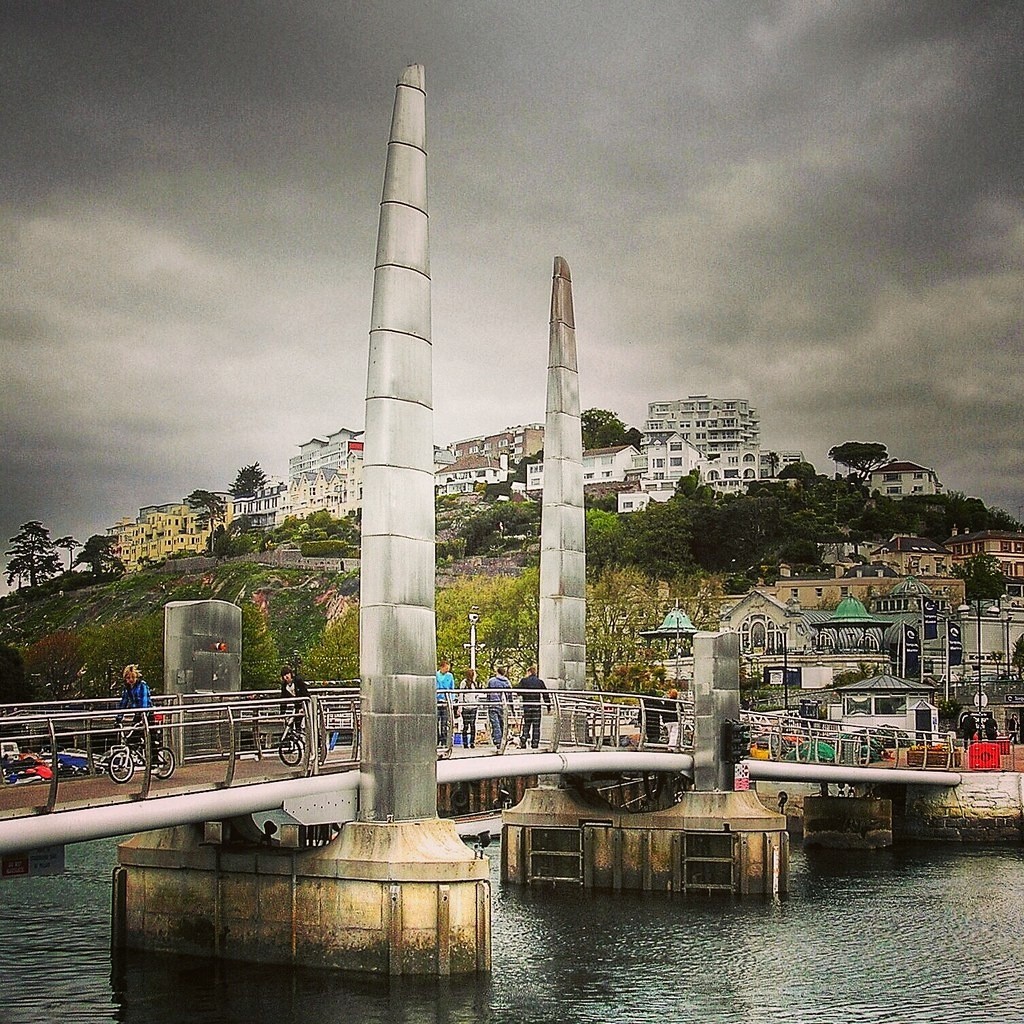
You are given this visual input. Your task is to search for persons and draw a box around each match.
[511,666,552,750]
[277,665,322,756]
[662,688,686,752]
[456,669,484,749]
[984,712,999,741]
[961,711,978,754]
[436,660,456,747]
[636,686,665,750]
[1006,714,1020,745]
[112,664,161,774]
[486,666,515,749]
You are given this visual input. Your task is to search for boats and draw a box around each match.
[0,742,115,786]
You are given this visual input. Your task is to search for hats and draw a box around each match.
[280,667,291,676]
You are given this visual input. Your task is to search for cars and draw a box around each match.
[454,692,519,716]
[748,738,786,762]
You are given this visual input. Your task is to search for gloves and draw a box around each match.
[113,718,121,728]
[133,715,142,723]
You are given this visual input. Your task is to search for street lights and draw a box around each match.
[462,606,485,683]
[779,624,793,709]
[958,596,999,742]
[1006,617,1012,679]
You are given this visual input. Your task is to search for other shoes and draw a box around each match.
[496,744,501,749]
[521,744,526,748]
[292,750,305,754]
[464,745,469,748]
[470,745,475,748]
[151,755,161,763]
[531,743,538,748]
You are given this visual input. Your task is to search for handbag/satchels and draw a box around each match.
[457,712,464,730]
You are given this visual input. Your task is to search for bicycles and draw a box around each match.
[279,709,327,767]
[108,712,175,784]
